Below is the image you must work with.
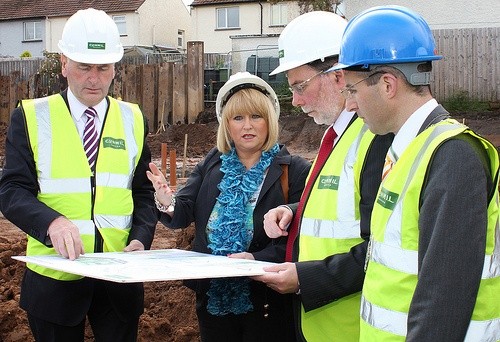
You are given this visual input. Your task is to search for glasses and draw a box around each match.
[340,71,397,98]
[289,70,325,94]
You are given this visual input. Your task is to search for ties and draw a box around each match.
[285,127,338,262]
[82,107,99,171]
[381,151,394,179]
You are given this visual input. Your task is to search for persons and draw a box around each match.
[147,72,312,342]
[250,11,395,342]
[322,4,500,342]
[0,9,159,342]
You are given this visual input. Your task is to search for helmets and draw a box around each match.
[323,4,442,73]
[58,8,124,65]
[269,10,349,76]
[216,72,280,123]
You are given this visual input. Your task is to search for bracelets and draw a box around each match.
[295,281,303,294]
[154,192,176,213]
